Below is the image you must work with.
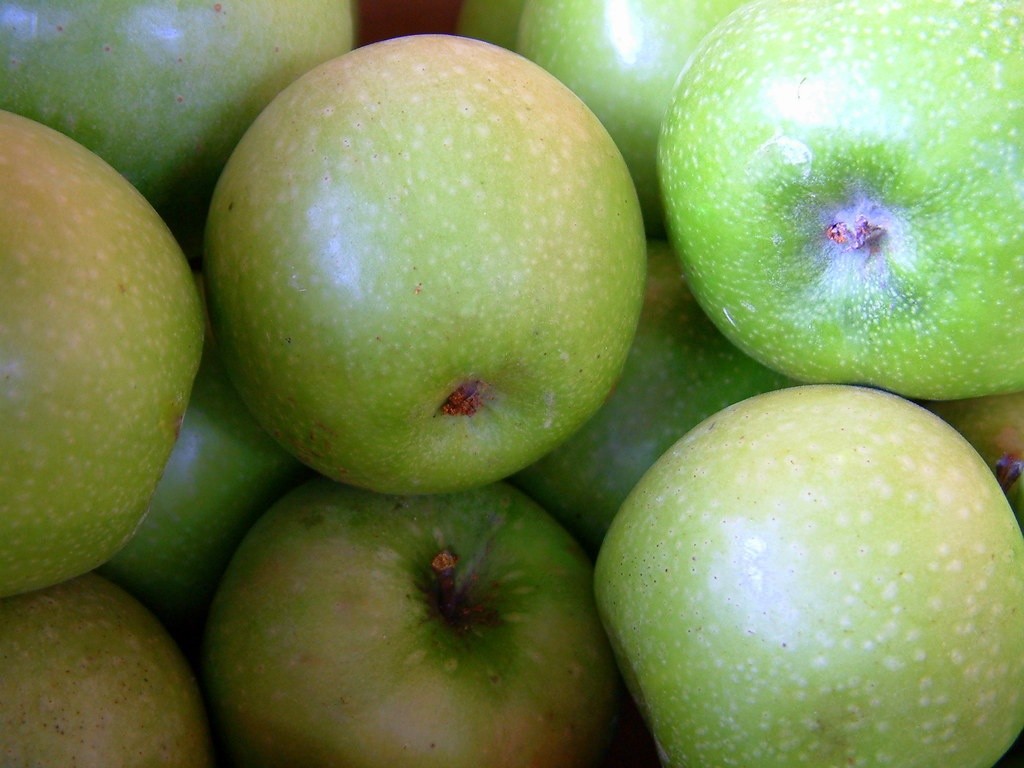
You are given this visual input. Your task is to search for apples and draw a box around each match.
[0,0,1024,768]
